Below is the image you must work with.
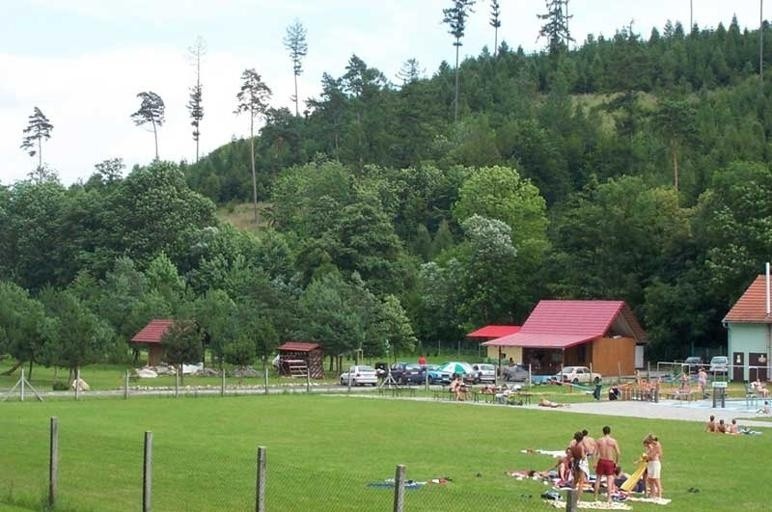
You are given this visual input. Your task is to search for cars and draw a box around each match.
[682,356,709,373]
[340,358,603,390]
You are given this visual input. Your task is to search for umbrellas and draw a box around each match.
[436,360,475,375]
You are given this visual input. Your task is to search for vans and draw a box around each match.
[708,356,729,375]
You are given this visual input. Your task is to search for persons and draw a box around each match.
[755,399,769,413]
[451,377,461,401]
[537,397,570,408]
[542,425,662,506]
[418,355,426,364]
[730,419,739,433]
[480,385,492,393]
[613,368,707,400]
[753,378,769,398]
[704,414,718,432]
[717,419,729,433]
